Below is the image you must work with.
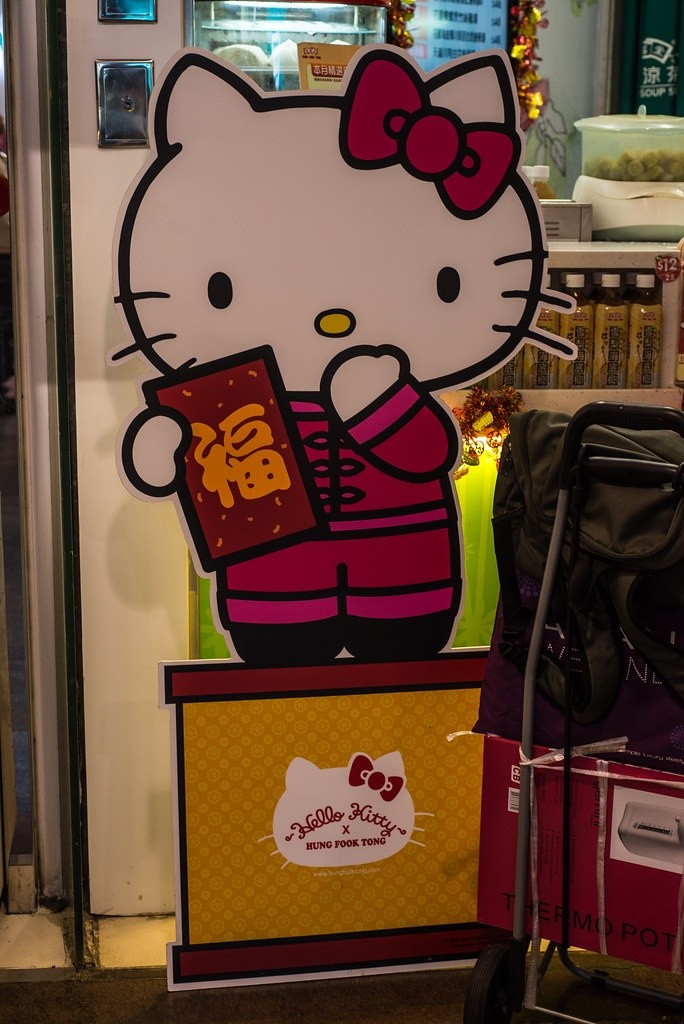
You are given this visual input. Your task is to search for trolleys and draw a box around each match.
[457,397,683,1024]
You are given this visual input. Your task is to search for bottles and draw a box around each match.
[588,272,629,388]
[624,272,662,389]
[523,166,534,184]
[534,165,556,199]
[557,272,595,389]
[524,273,560,389]
[488,346,523,389]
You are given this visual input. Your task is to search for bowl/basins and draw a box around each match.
[573,104,684,182]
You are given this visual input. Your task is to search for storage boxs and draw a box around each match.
[477,736,684,975]
